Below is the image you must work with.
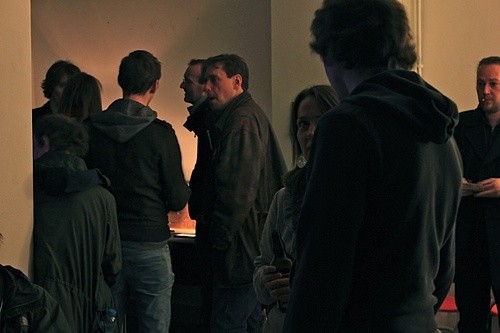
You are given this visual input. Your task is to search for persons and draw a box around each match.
[0,233,71,333]
[202,54,288,331]
[88,51,190,333]
[57,72,101,120]
[32,60,80,119]
[453,56,500,333]
[180,60,217,333]
[32,114,124,333]
[253,85,341,333]
[281,0,464,333]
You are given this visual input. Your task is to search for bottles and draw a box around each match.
[270,230,293,313]
[106,309,116,323]
